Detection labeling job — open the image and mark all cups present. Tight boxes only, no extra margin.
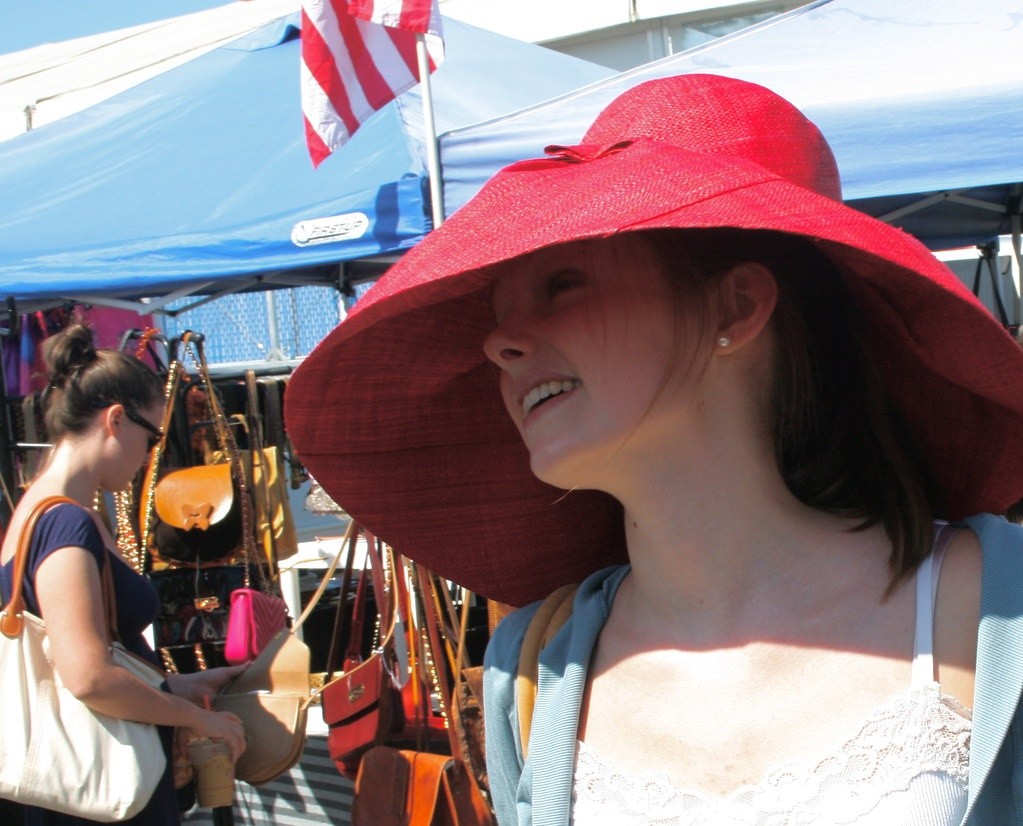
[185,739,233,809]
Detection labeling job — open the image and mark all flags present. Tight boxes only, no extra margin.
[300,0,446,169]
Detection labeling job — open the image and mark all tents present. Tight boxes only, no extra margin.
[3,8,619,665]
[436,4,1022,259]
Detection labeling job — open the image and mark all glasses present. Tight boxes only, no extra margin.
[92,400,163,455]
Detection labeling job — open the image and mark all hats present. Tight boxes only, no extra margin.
[282,72,1023,611]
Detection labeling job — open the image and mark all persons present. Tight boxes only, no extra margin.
[0,323,259,826]
[482,76,1023,826]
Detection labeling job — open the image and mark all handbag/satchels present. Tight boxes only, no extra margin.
[89,329,497,826]
[0,495,169,824]
